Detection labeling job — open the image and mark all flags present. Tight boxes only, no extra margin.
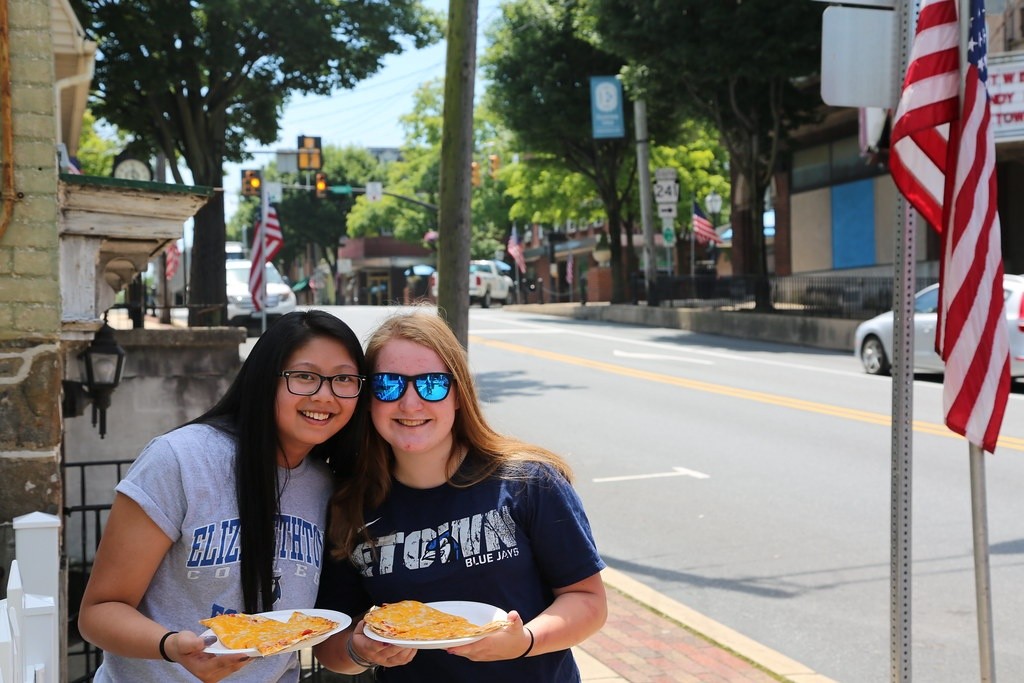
[507,216,526,275]
[166,240,181,280]
[566,247,574,285]
[691,197,724,245]
[248,167,283,311]
[889,1,1011,456]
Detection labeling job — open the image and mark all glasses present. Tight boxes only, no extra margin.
[372,371,455,403]
[275,364,367,399]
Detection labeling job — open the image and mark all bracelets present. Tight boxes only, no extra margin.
[518,625,535,660]
[155,630,180,663]
[345,632,379,670]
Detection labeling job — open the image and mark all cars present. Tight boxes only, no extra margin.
[853,274,1024,392]
[225,258,297,326]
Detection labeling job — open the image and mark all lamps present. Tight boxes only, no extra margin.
[62,312,125,439]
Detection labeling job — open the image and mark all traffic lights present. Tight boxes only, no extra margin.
[315,173,327,198]
[242,170,261,195]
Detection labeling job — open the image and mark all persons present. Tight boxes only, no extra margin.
[313,313,608,683]
[77,310,371,683]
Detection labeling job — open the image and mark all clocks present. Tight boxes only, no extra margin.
[112,158,154,181]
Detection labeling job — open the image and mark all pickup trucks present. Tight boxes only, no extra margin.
[430,260,515,308]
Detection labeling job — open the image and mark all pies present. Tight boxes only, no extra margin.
[364,600,514,640]
[199,611,339,659]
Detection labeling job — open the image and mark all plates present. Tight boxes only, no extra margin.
[199,609,352,657]
[362,601,507,649]
